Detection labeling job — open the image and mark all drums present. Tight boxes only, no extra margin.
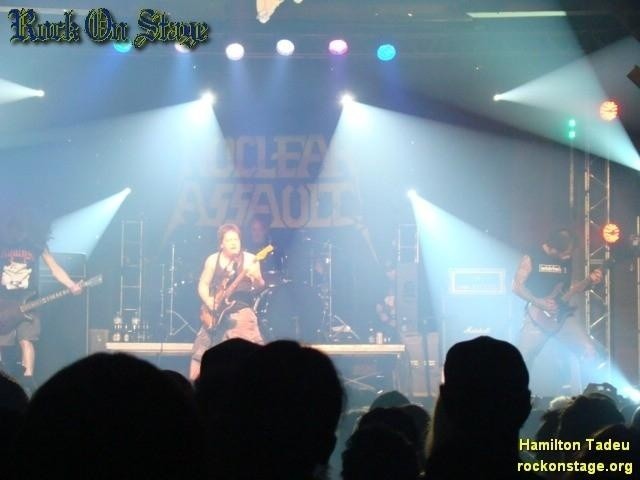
[254,280,325,341]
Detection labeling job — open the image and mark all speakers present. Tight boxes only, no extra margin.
[34,280,88,385]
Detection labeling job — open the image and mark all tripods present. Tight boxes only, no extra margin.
[158,258,361,343]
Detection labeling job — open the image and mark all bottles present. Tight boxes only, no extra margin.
[130,311,140,331]
[112,311,124,330]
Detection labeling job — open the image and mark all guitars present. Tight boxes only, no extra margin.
[201,245,273,330]
[0,273,103,336]
[527,256,616,335]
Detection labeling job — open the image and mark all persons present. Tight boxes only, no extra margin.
[3,333,638,479]
[512,228,606,384]
[189,223,266,381]
[372,261,398,393]
[0,205,83,377]
